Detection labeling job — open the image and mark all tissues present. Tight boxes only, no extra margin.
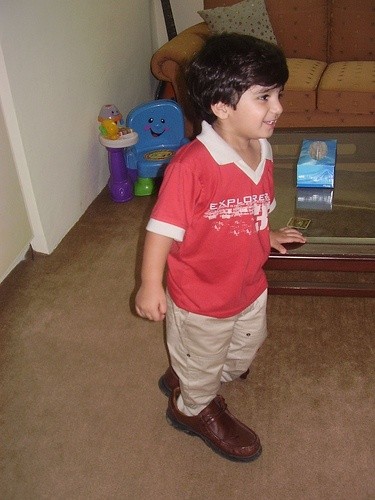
[296,138,337,188]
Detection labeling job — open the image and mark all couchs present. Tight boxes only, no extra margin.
[149,0,375,138]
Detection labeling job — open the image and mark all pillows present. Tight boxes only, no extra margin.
[197,0,278,46]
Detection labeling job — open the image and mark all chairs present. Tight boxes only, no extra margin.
[123,98,193,196]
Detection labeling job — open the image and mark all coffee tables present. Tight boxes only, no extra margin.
[240,128,375,380]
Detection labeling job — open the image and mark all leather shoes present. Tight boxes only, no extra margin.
[166,385,263,461]
[159,369,183,395]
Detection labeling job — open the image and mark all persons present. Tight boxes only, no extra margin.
[135,33,307,462]
